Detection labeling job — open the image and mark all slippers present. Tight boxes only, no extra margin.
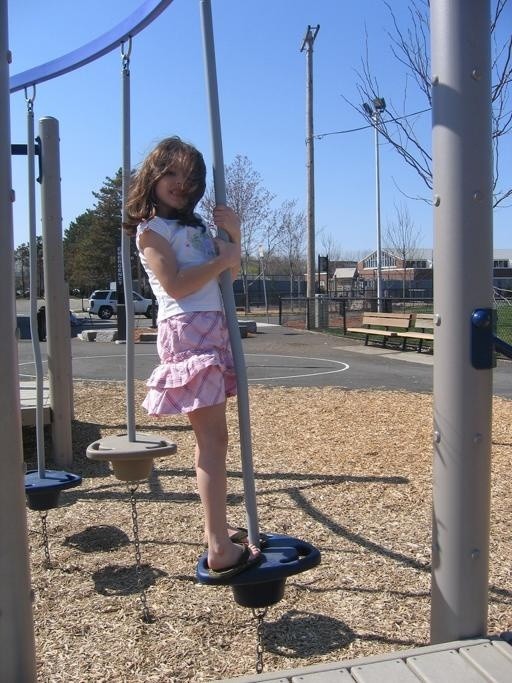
[204,526,269,579]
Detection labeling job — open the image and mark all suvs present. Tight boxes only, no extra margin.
[86,287,159,319]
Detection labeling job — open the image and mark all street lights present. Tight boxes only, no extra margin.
[363,96,387,314]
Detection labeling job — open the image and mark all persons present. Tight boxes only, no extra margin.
[120,135,269,579]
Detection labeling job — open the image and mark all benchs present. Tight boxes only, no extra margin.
[346,312,412,351]
[397,314,434,353]
